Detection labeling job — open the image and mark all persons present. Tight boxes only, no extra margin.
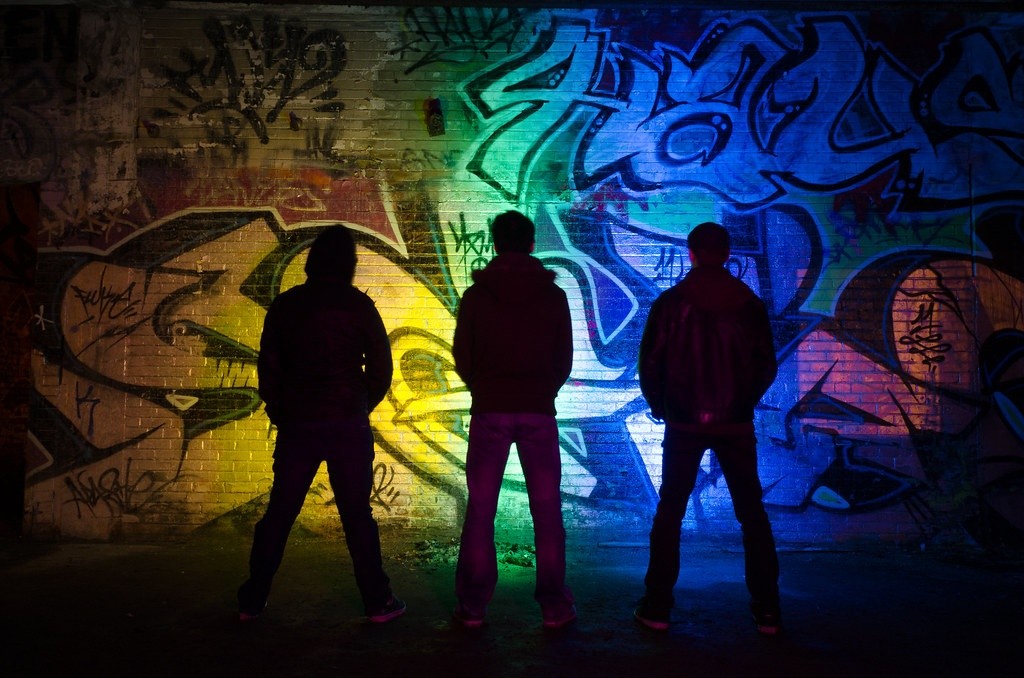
[451,209,578,631]
[633,222,786,635]
[236,222,407,623]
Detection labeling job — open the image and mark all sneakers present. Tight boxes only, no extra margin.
[368,595,407,624]
[237,601,267,622]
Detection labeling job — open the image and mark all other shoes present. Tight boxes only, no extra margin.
[453,611,484,628]
[753,615,782,633]
[633,601,669,631]
[543,605,576,628]
